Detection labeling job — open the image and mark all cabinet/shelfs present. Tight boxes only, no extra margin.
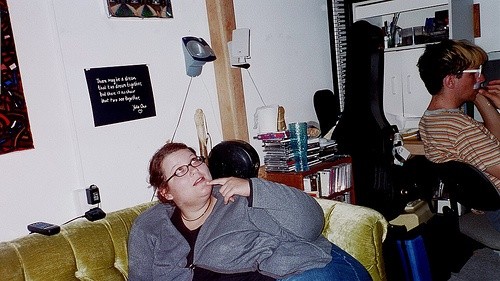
[352,0,474,132]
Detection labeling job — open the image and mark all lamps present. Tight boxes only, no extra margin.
[182,36,217,78]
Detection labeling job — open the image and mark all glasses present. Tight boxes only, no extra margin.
[458,65,483,78]
[164,156,206,184]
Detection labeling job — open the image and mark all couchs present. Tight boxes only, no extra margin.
[0,196,388,281]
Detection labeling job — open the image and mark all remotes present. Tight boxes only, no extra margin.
[27,222,61,236]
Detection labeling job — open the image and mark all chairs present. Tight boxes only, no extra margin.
[416,154,500,250]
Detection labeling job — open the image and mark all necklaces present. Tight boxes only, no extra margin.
[181,197,211,221]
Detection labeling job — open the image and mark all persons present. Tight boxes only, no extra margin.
[416,39,500,234]
[127,142,373,281]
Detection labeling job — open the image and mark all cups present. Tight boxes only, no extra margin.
[289,122,310,172]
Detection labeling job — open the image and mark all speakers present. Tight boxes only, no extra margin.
[232,28,250,68]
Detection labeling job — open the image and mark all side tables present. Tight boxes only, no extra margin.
[267,154,355,204]
